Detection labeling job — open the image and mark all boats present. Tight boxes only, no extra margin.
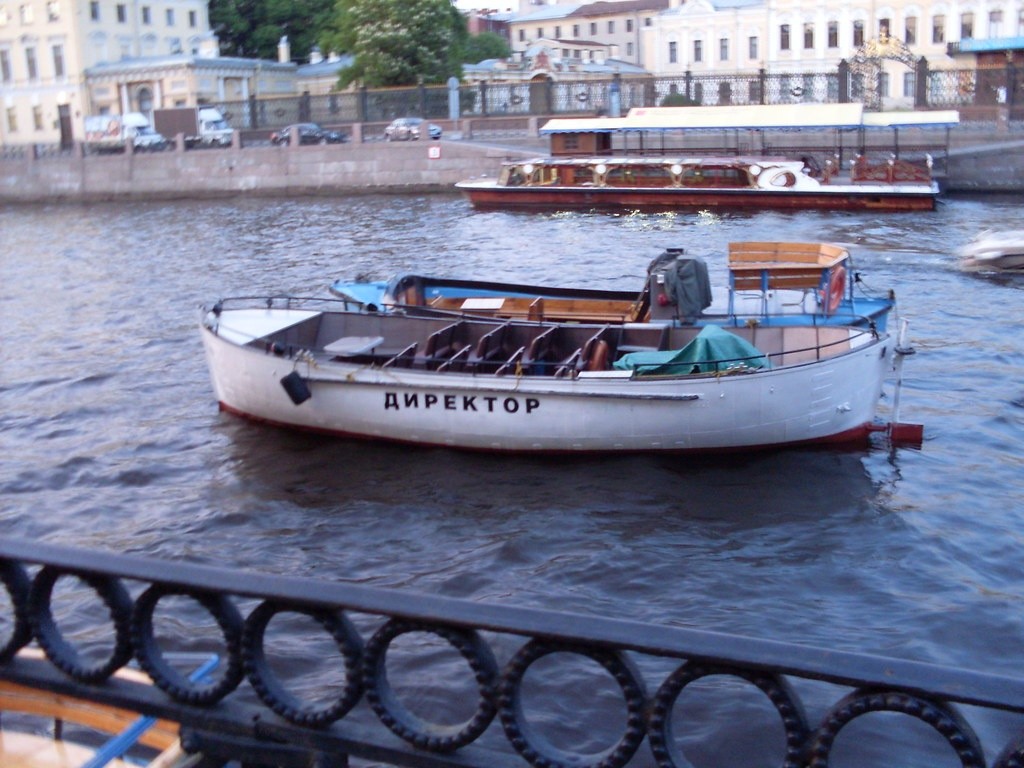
[195,296,923,450]
[328,240,896,333]
[453,104,960,212]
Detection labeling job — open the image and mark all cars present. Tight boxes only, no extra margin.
[272,124,346,147]
[385,117,442,141]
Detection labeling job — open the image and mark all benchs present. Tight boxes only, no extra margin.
[528,296,545,321]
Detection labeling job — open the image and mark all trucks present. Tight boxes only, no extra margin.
[84,108,235,150]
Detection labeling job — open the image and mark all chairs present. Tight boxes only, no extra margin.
[382,320,670,378]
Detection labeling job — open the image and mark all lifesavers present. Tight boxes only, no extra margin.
[817,266,846,311]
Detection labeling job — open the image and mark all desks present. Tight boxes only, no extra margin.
[460,298,506,317]
[324,335,384,365]
